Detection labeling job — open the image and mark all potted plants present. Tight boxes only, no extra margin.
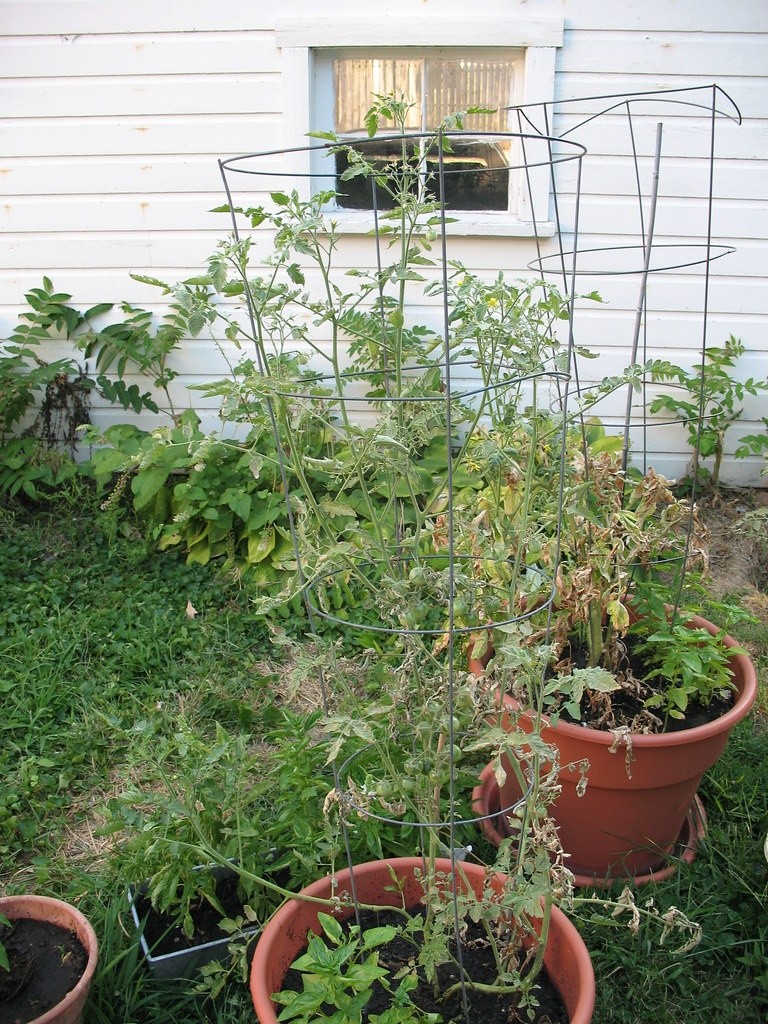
[467,340,768,890]
[0,893,100,1024]
[130,87,599,1024]
[88,705,424,985]
